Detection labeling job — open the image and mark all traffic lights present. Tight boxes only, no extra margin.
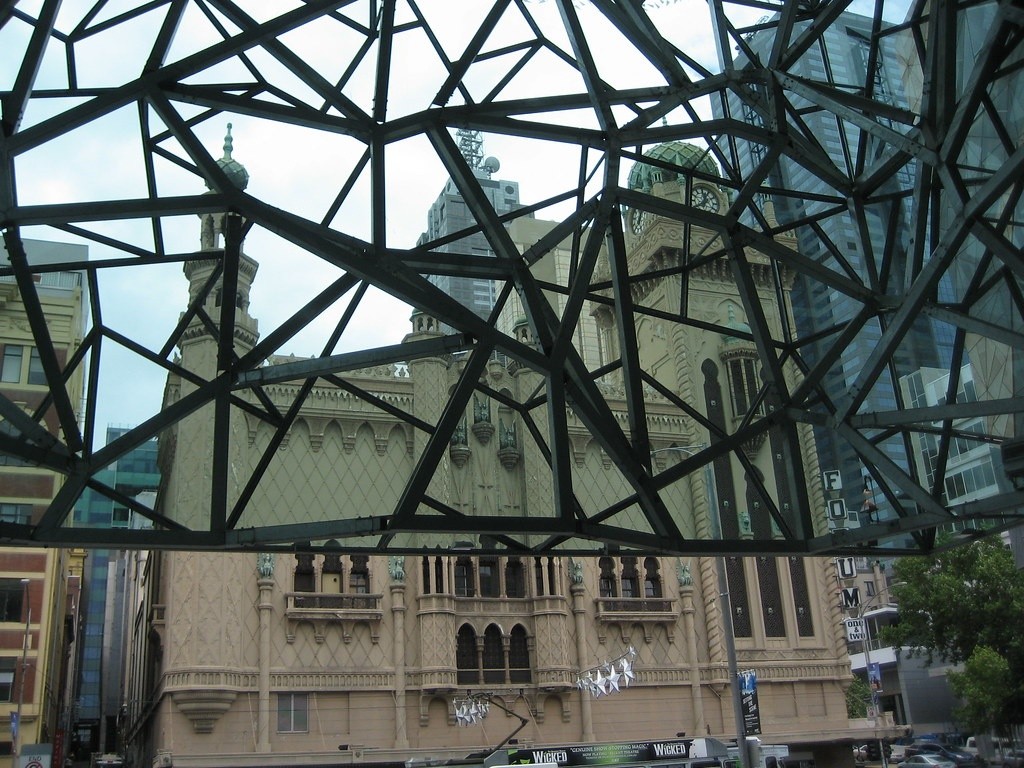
[883,740,892,759]
[866,740,879,761]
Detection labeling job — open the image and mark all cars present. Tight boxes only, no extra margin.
[853,744,906,763]
[897,753,957,768]
[966,739,1024,768]
[904,743,984,768]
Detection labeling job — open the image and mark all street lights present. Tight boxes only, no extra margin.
[857,580,908,768]
[10,578,34,768]
